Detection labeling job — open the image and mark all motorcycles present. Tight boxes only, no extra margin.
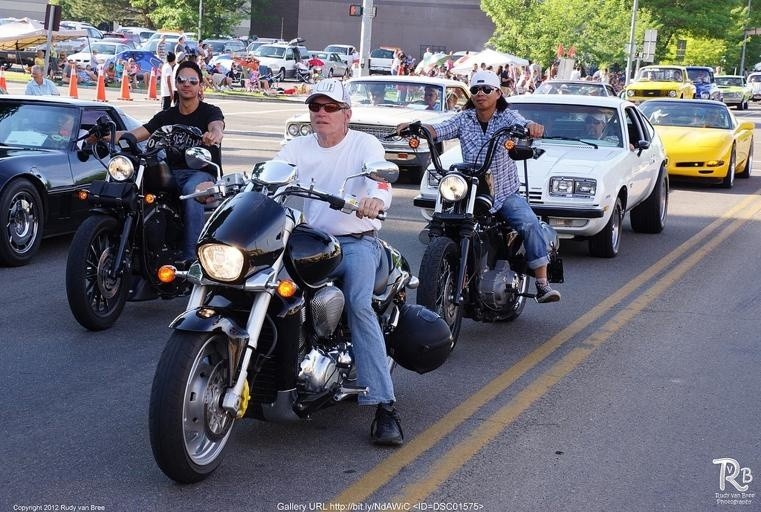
[63,116,254,331]
[382,116,564,354]
[146,136,455,485]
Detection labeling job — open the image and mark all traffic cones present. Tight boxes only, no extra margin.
[145,67,159,99]
[116,70,134,101]
[0,66,6,89]
[93,66,109,103]
[68,64,79,96]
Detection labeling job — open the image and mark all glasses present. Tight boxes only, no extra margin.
[176,75,198,85]
[308,103,346,112]
[587,119,600,124]
[470,85,493,95]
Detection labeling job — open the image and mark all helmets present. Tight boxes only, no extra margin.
[385,303,452,375]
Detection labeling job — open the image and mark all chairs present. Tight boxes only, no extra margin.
[607,124,617,135]
[80,123,96,132]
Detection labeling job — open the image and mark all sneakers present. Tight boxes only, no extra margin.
[373,406,402,446]
[535,281,560,303]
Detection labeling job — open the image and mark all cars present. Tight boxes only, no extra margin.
[413,95,673,258]
[523,64,761,188]
[281,68,474,186]
[0,17,411,89]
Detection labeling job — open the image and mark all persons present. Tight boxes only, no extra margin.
[24,34,609,447]
[707,108,720,122]
[674,71,680,80]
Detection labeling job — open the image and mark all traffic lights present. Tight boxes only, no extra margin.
[350,5,361,15]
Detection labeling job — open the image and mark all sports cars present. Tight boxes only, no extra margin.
[0,94,148,268]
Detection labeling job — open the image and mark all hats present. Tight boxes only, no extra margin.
[304,79,350,107]
[587,110,606,124]
[175,61,202,82]
[470,70,499,89]
